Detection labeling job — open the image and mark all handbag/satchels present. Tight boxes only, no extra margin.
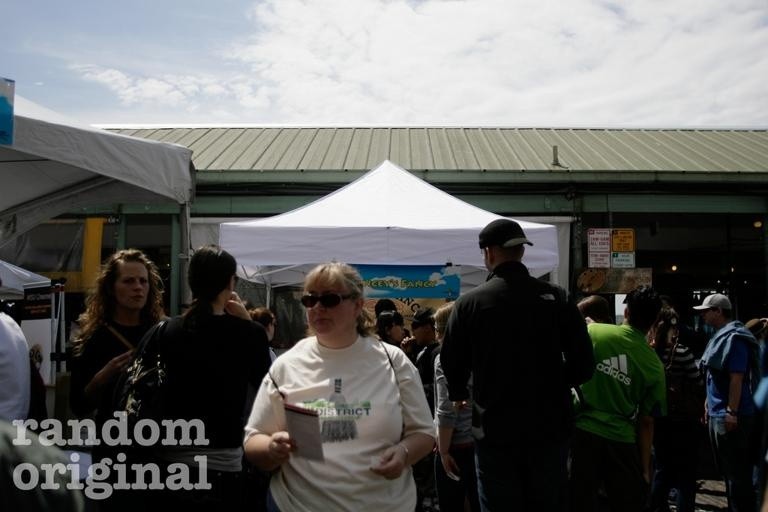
[114,353,169,422]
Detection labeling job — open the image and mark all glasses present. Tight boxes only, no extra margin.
[301,293,351,308]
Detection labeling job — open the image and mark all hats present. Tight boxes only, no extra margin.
[403,307,436,323]
[479,220,533,248]
[745,318,768,335]
[693,293,732,310]
